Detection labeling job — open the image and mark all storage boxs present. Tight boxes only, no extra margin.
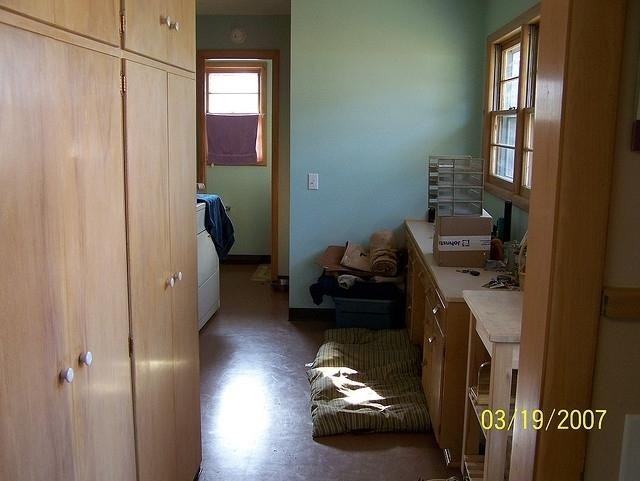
[332,296,395,330]
[433,208,493,267]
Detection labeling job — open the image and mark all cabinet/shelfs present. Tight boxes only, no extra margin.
[461,290,524,481]
[0,0,201,481]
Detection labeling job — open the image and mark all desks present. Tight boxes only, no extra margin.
[403,220,520,468]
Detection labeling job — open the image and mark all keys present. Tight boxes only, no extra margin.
[456,267,480,277]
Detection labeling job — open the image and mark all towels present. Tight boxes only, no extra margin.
[206,114,258,165]
